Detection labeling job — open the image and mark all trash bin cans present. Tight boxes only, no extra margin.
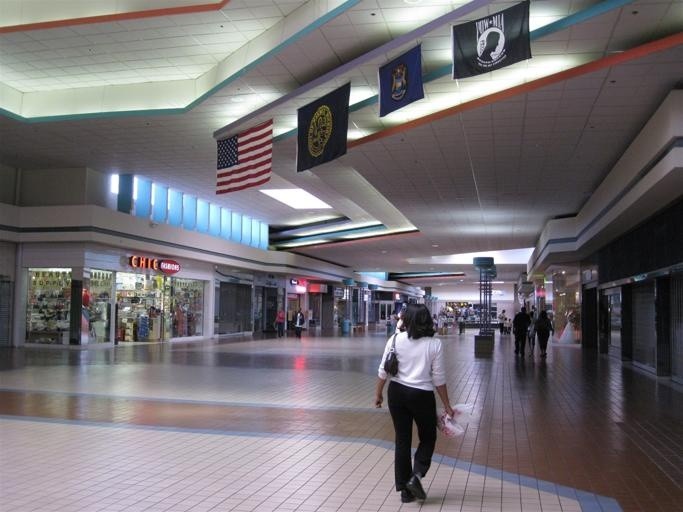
[387,324,395,338]
[459,322,466,336]
[341,320,350,337]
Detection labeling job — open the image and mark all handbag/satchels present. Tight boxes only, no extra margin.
[384,333,398,376]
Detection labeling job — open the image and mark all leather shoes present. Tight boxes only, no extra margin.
[401,475,427,503]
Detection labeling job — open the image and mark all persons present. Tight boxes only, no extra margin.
[149,306,160,317]
[498,310,512,335]
[294,308,304,339]
[373,303,454,503]
[513,307,554,357]
[275,308,285,337]
